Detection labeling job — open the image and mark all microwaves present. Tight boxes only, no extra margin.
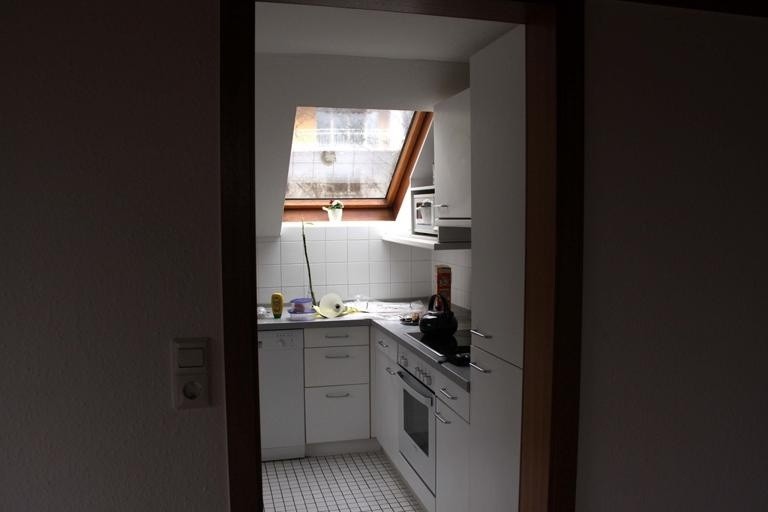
[411,192,439,238]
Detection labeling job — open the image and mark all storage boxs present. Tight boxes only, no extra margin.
[434,264,452,310]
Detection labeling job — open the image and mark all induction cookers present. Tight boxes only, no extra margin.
[405,327,471,357]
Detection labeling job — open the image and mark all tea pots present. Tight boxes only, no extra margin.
[420,294,460,339]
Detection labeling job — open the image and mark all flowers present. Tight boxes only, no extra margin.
[322,199,345,210]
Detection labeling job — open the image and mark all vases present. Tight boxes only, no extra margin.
[327,209,343,223]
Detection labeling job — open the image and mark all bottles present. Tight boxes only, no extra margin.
[397,301,424,326]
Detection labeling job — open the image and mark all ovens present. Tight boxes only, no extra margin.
[393,363,437,511]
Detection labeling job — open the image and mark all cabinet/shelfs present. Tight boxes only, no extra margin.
[431,89,471,232]
[253,326,305,463]
[434,373,470,511]
[370,328,399,465]
[303,322,370,448]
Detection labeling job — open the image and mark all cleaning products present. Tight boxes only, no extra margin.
[271,293,284,318]
